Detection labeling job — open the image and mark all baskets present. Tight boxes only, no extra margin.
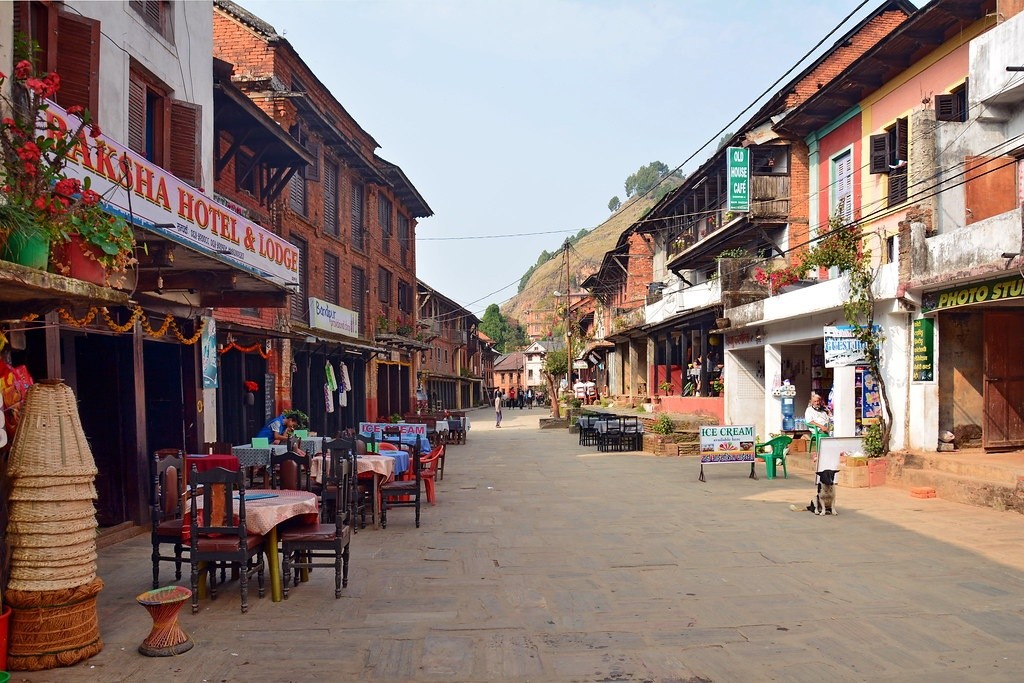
[7,572,96,591]
[9,499,97,522]
[6,515,99,534]
[13,551,97,567]
[7,528,96,547]
[9,482,98,500]
[12,539,96,561]
[10,561,97,581]
[11,475,96,487]
[6,383,98,476]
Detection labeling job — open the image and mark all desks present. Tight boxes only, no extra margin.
[378,449,408,480]
[593,420,645,451]
[375,436,431,454]
[310,455,397,530]
[576,416,599,444]
[299,436,332,454]
[186,455,239,485]
[182,489,319,601]
[232,443,287,489]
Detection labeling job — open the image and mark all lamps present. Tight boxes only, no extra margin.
[407,350,426,363]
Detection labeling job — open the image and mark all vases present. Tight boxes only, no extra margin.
[1,231,50,272]
[378,328,387,334]
[666,390,672,396]
[653,398,660,404]
[52,233,108,286]
[719,392,724,397]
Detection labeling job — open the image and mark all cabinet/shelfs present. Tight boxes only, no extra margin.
[810,344,834,391]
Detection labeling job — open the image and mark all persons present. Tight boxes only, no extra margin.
[494,386,507,428]
[560,378,568,395]
[572,378,596,399]
[804,395,834,436]
[527,386,534,410]
[509,387,515,411]
[517,386,524,410]
[256,415,298,446]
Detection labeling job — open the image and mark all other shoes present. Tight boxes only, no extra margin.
[496,425,501,428]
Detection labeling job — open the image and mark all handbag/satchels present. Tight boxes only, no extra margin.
[532,396,535,400]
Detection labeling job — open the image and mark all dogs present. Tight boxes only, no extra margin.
[790,470,840,516]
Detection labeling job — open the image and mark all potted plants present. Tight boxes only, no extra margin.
[864,422,886,488]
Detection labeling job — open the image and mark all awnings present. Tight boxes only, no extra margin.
[580,242,631,306]
[214,74,318,208]
[665,215,788,286]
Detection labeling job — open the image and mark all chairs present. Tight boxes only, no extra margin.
[151,408,471,614]
[751,436,793,479]
[804,420,828,454]
[577,386,597,404]
[579,410,639,451]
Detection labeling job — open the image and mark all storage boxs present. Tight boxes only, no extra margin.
[838,465,869,488]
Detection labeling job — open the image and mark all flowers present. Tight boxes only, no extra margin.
[0,29,150,287]
[376,307,424,341]
[652,379,674,398]
[711,376,725,391]
